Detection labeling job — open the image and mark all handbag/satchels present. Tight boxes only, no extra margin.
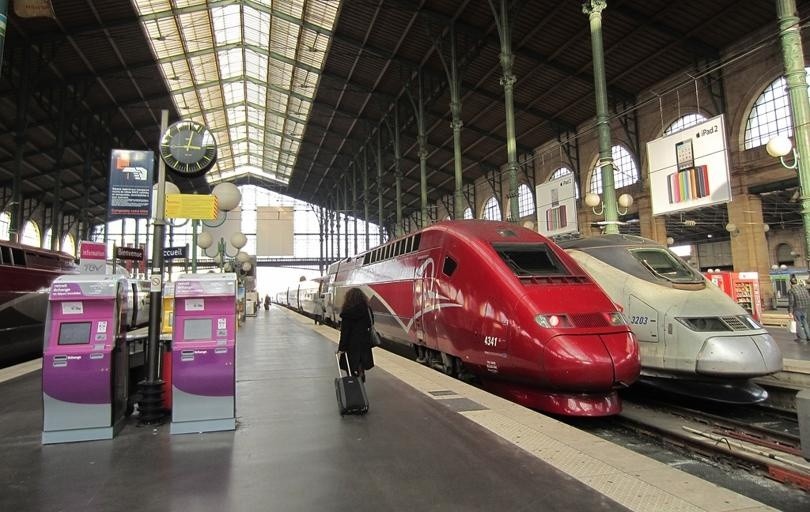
[322,306,326,313]
[368,307,381,348]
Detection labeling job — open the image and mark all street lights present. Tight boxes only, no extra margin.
[151,182,243,274]
[214,250,252,280]
[195,230,248,273]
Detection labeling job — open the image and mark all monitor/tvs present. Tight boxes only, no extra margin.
[58,321,92,344]
[184,319,212,340]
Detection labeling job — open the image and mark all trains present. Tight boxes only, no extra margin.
[265,219,641,418]
[550,234,783,405]
[0,239,167,369]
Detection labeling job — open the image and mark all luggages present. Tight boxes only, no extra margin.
[264,304,269,310]
[332,351,369,417]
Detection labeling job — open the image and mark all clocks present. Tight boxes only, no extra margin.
[157,120,219,177]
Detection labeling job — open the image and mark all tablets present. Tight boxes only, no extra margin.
[674,138,694,172]
[550,188,559,207]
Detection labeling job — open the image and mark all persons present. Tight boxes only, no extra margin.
[313,295,327,326]
[787,277,810,342]
[337,288,374,383]
[265,293,272,309]
